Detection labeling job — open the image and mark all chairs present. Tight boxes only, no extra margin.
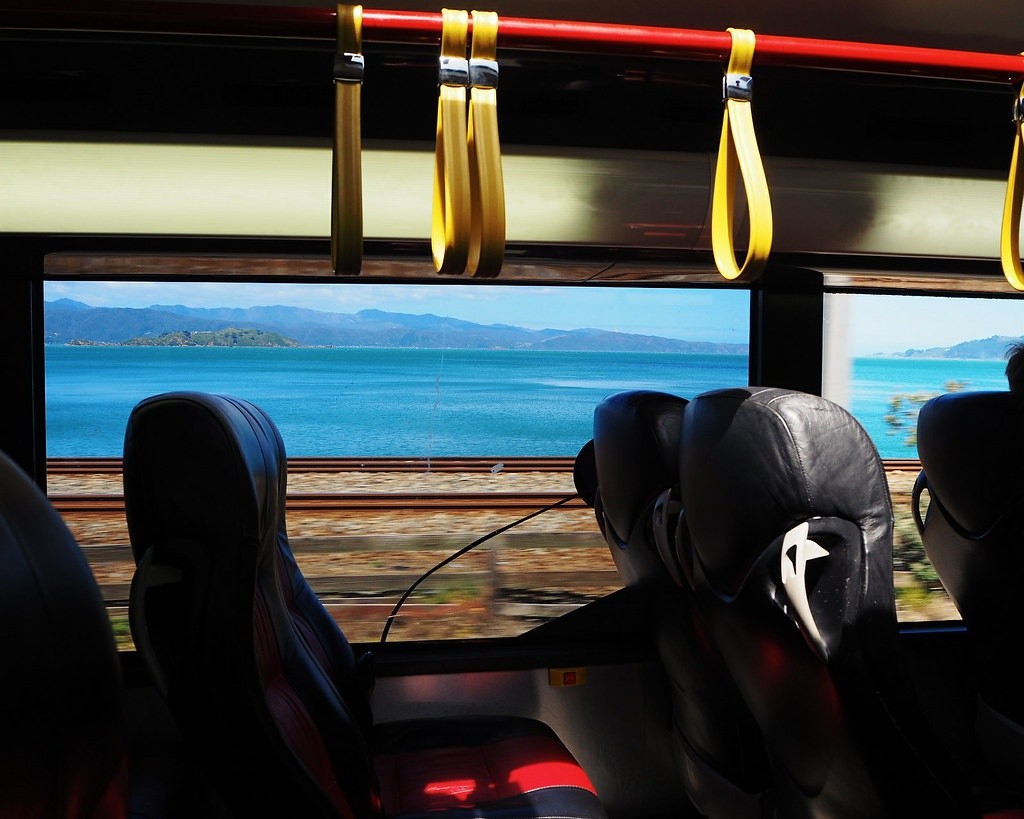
[0,390,1024,819]
